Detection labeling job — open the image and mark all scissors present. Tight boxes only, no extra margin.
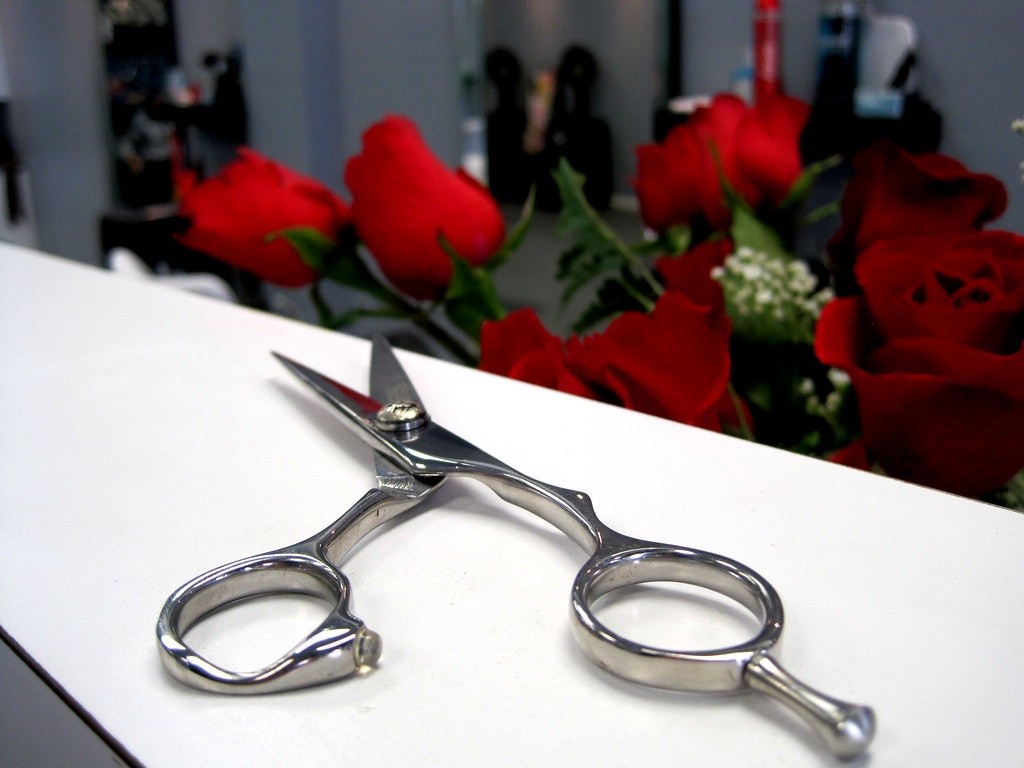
[156,329,877,763]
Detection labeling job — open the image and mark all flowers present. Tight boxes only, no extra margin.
[182,84,1024,523]
